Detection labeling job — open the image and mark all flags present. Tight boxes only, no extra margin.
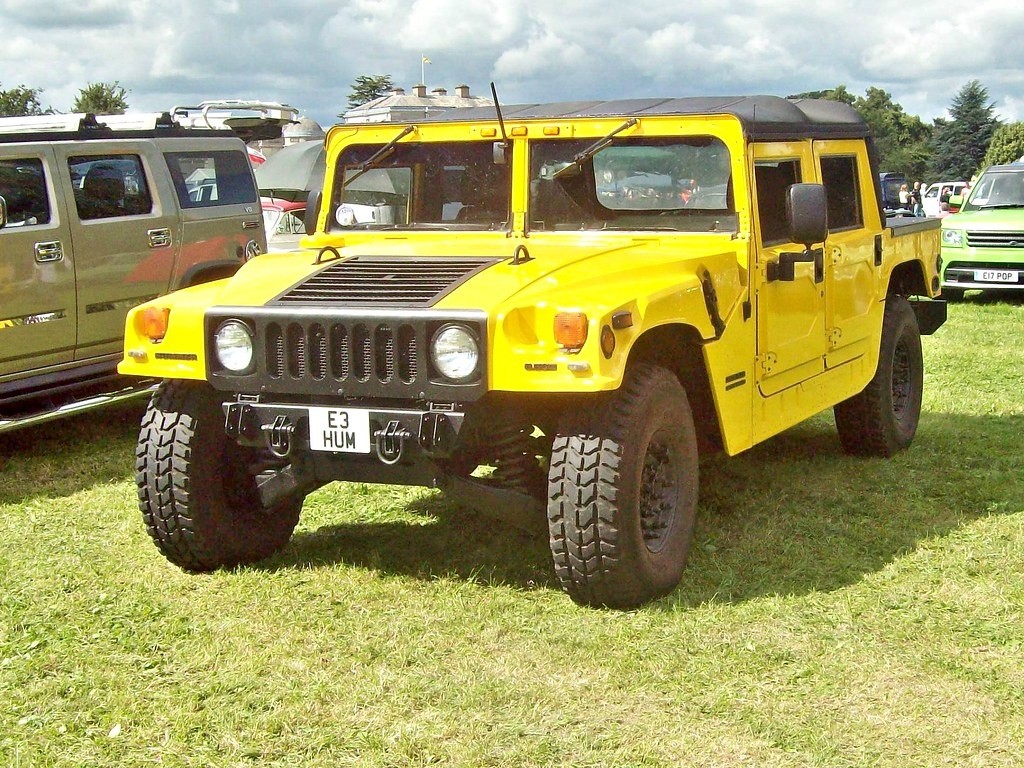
[423,57,432,63]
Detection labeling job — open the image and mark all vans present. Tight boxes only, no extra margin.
[880,172,975,218]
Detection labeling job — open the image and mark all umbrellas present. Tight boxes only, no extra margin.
[254,139,412,203]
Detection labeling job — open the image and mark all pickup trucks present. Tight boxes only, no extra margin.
[114,100,946,612]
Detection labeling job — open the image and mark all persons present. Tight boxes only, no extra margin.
[899,184,913,210]
[961,181,972,202]
[690,179,701,193]
[911,182,926,217]
[940,189,949,203]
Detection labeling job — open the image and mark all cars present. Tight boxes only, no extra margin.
[938,156,1024,303]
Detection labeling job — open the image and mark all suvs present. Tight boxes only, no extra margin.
[0,103,409,426]
